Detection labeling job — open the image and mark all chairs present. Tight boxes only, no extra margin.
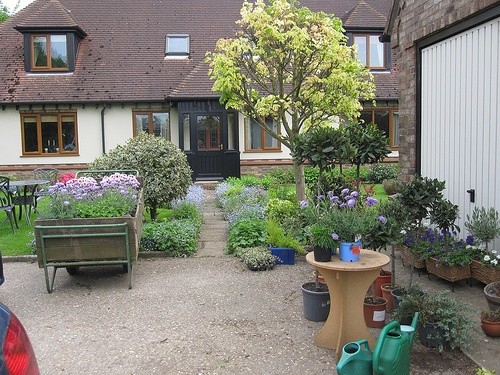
[0,175,19,234]
[33,167,58,213]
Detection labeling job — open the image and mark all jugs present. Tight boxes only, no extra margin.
[335,339,373,375]
[372,312,419,375]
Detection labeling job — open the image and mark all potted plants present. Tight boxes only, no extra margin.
[281,119,393,322]
[363,215,407,329]
[389,286,488,354]
[385,172,462,325]
[262,218,307,265]
[480,306,500,338]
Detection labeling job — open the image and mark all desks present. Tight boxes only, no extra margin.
[306,248,390,365]
[0,180,49,227]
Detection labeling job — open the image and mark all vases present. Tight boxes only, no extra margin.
[338,240,363,262]
[312,243,333,263]
[32,169,146,295]
[468,257,500,288]
[424,254,472,294]
[363,297,388,328]
[370,269,391,298]
[391,241,426,277]
[483,280,500,312]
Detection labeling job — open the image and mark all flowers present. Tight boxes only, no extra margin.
[296,183,389,249]
[394,198,500,269]
[34,172,142,220]
